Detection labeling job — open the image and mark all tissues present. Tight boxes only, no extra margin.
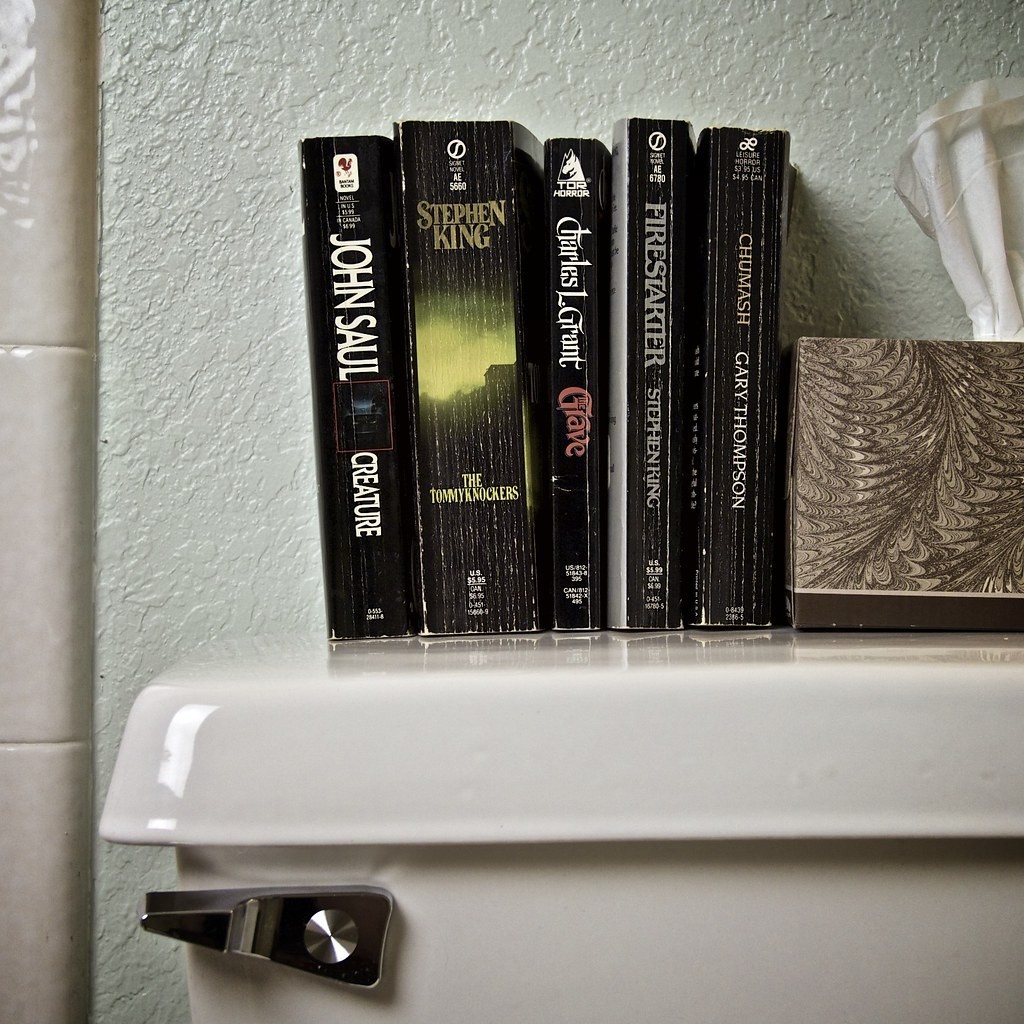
[781,76,1024,633]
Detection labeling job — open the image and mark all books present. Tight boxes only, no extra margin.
[302,117,791,640]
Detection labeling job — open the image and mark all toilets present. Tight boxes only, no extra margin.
[91,630,1024,1024]
